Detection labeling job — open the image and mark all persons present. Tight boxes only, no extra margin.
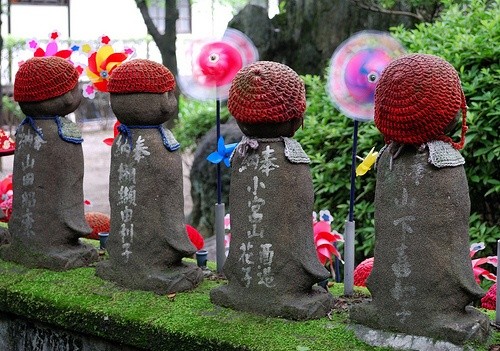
[209,59,336,321]
[94,58,205,295]
[8,56,99,271]
[348,53,490,346]
